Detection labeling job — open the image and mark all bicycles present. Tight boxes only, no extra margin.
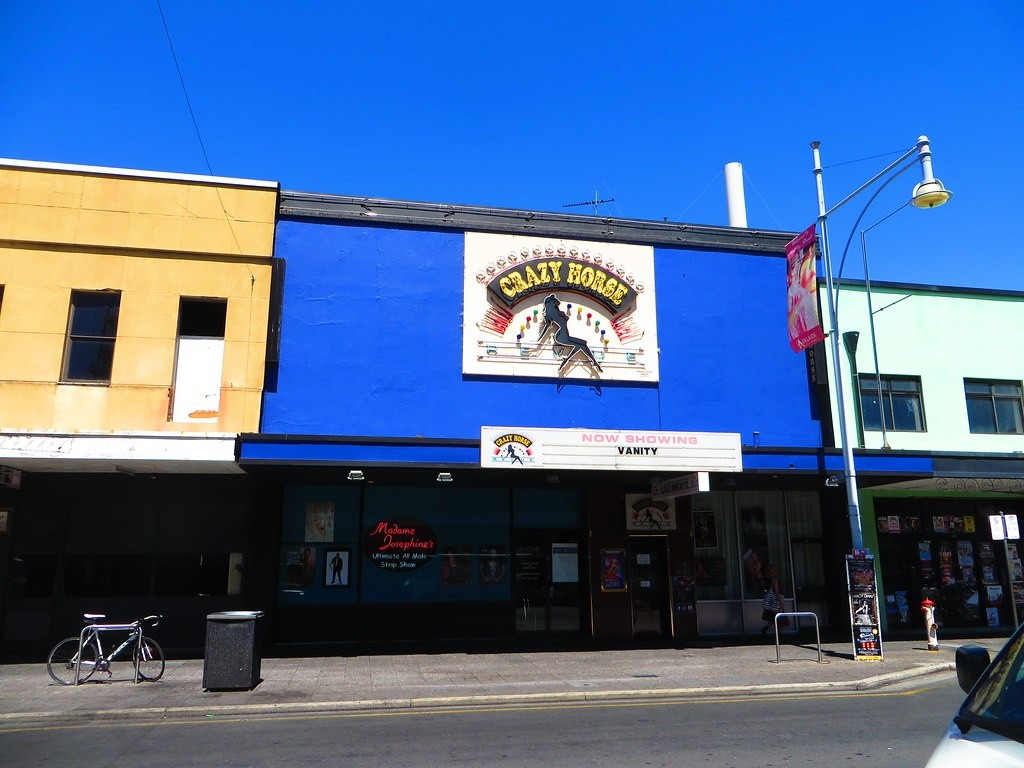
[44,612,168,684]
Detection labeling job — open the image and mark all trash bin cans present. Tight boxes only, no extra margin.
[202,611,264,689]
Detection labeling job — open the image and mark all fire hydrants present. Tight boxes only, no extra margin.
[917,597,941,651]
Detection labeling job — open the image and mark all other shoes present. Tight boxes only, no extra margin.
[761,628,768,638]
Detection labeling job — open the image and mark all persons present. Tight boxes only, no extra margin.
[760,566,790,641]
[695,511,767,596]
[299,515,505,585]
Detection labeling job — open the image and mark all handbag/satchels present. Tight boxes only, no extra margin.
[762,584,785,613]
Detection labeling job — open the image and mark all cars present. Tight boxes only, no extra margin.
[926,621,1024,768]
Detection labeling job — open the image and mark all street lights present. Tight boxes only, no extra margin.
[807,133,950,547]
